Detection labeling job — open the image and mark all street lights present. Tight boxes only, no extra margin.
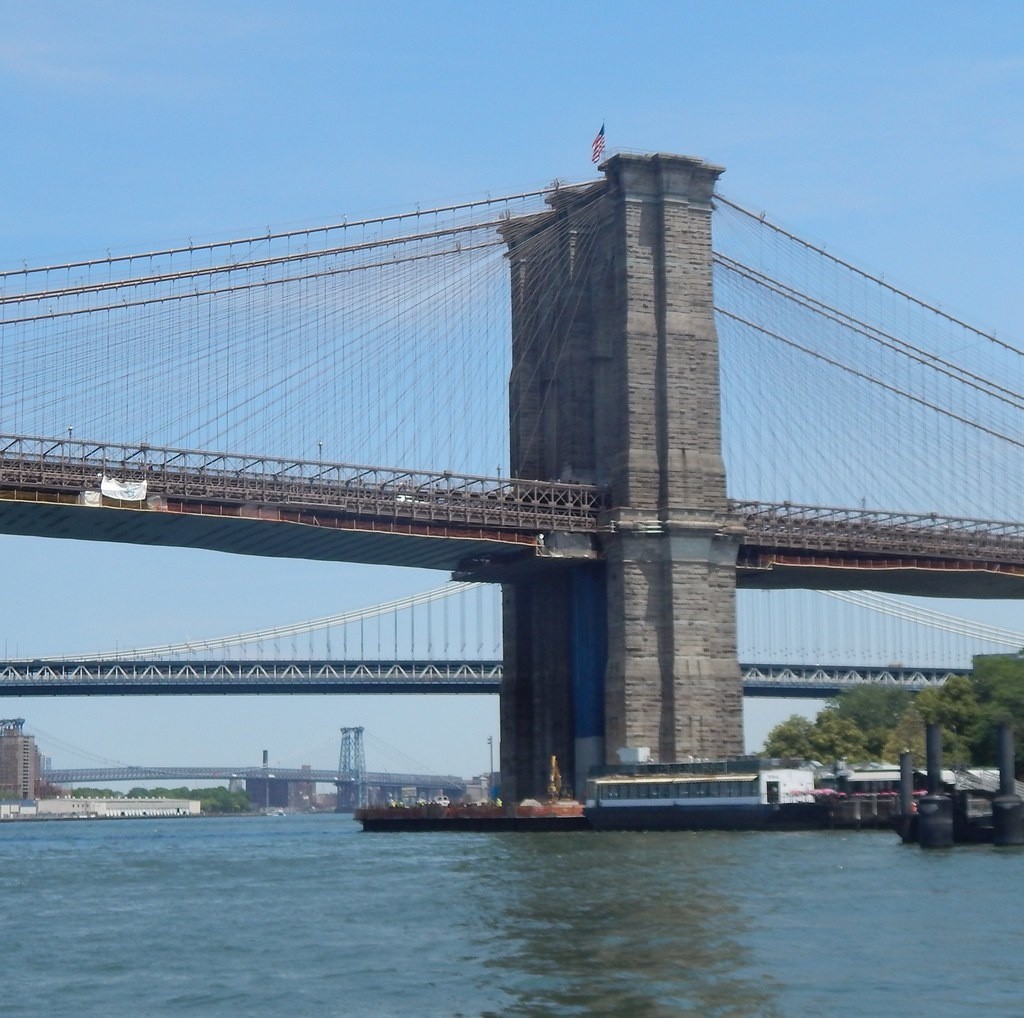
[318,442,323,484]
[68,425,75,463]
[497,465,503,488]
[861,496,867,523]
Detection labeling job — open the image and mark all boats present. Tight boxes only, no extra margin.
[580,766,840,832]
[264,808,287,817]
[351,800,589,834]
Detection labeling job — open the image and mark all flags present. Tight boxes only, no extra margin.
[592,123,604,164]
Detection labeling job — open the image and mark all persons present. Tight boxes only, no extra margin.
[137,457,144,471]
[147,460,154,471]
[770,787,778,804]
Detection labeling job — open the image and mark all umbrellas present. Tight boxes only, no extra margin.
[785,787,838,804]
[839,791,846,798]
[851,790,928,797]
